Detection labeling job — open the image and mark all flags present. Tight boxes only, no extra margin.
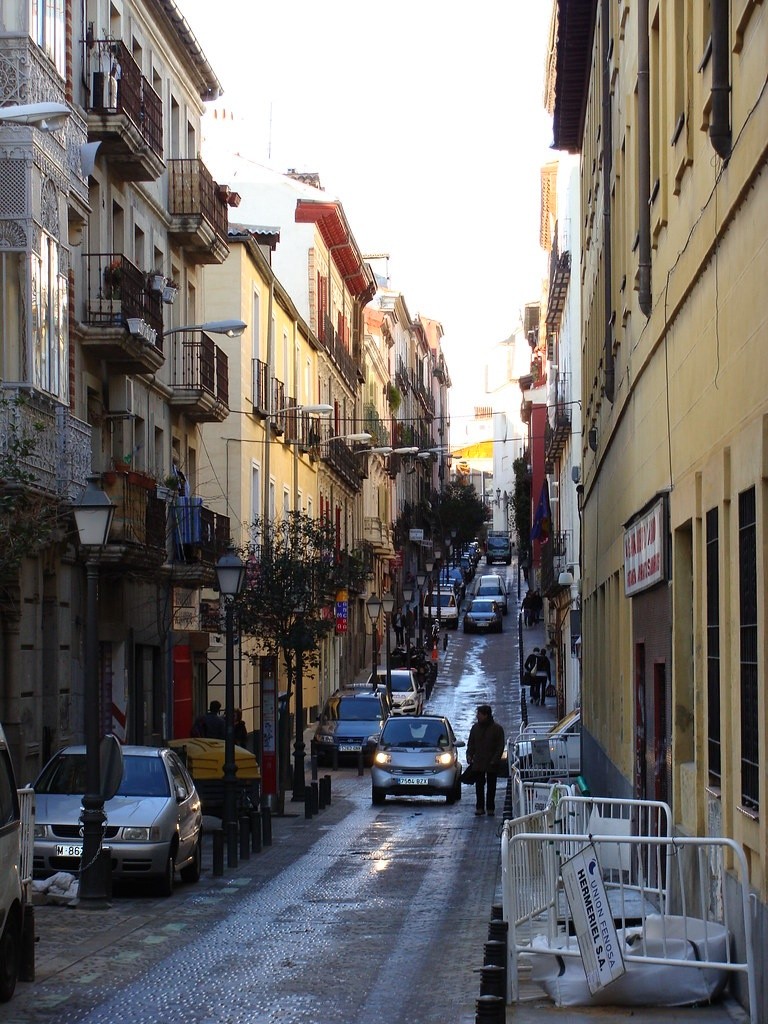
[531,478,553,542]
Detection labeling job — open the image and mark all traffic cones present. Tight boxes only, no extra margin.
[432,641,439,660]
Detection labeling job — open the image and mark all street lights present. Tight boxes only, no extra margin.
[425,558,435,645]
[366,592,382,689]
[285,559,313,802]
[402,582,414,670]
[433,547,442,627]
[450,529,456,568]
[69,473,119,909]
[381,590,395,709]
[444,537,451,583]
[416,570,427,646]
[216,547,250,868]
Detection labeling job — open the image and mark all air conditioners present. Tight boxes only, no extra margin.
[89,71,117,113]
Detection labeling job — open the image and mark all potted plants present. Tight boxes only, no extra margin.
[156,474,180,503]
[147,269,182,304]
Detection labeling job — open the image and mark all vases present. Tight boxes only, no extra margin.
[127,471,156,489]
[227,192,241,207]
[220,184,231,198]
[85,299,129,326]
[103,471,116,486]
[115,463,131,472]
[127,318,158,346]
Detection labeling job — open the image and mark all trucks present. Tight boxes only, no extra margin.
[484,530,515,565]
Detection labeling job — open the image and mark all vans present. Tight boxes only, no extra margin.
[470,575,510,615]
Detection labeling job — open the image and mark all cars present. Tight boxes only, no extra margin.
[504,712,580,783]
[18,745,202,894]
[368,715,465,804]
[0,722,22,1001]
[366,670,424,716]
[433,541,482,604]
[462,599,502,633]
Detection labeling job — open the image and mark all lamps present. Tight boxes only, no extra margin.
[418,447,448,452]
[437,453,462,459]
[162,321,248,338]
[0,101,71,133]
[417,453,430,458]
[316,433,372,444]
[265,403,333,421]
[353,447,393,457]
[382,447,419,456]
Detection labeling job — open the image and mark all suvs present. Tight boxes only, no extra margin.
[313,688,391,765]
[422,589,459,629]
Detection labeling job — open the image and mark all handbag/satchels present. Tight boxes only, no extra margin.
[523,672,531,686]
[545,682,557,697]
[531,658,537,676]
[460,763,476,785]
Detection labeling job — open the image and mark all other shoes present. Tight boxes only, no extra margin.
[541,703,545,706]
[475,809,486,815]
[536,700,539,706]
[530,697,533,704]
[488,810,495,816]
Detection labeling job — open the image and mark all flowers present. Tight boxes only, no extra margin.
[95,259,127,301]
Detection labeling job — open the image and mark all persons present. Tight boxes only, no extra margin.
[423,722,441,745]
[525,647,550,706]
[521,589,542,628]
[466,705,504,816]
[193,700,248,749]
[391,606,405,647]
